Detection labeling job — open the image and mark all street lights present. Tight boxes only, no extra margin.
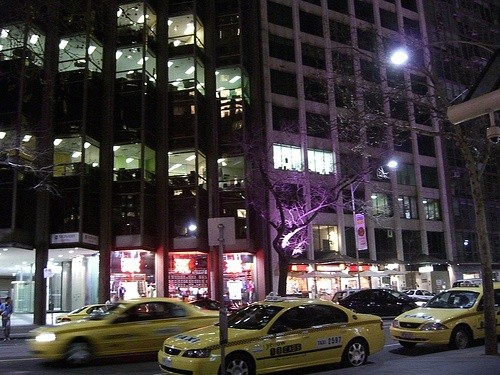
[351,160,397,289]
[390,40,495,66]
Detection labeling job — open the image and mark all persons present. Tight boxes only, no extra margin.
[0,297,14,342]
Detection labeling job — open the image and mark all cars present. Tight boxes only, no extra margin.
[337,289,418,316]
[157,292,385,375]
[332,278,482,303]
[56,304,106,324]
[389,282,500,350]
[24,297,230,366]
